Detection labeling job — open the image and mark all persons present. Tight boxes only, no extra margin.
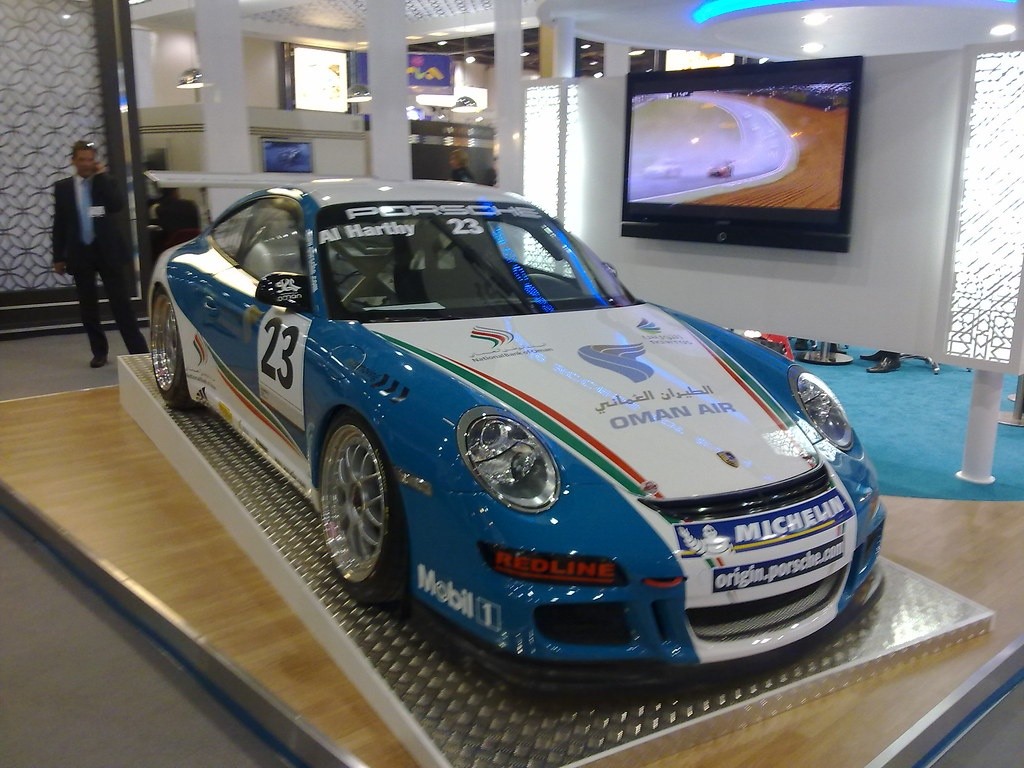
[860,350,901,373]
[447,148,479,184]
[52,140,150,369]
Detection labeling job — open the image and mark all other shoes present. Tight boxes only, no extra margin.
[90,355,107,367]
[795,343,811,350]
[831,349,846,354]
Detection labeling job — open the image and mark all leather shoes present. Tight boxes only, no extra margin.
[860,350,893,361]
[867,353,900,373]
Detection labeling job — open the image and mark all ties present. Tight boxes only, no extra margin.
[81,180,96,245]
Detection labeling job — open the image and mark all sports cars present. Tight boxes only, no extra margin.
[148,176,890,684]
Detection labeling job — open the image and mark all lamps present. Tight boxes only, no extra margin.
[347,83,374,103]
[175,37,204,90]
[451,96,479,114]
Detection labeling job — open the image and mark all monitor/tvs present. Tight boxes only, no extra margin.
[621,56,865,253]
